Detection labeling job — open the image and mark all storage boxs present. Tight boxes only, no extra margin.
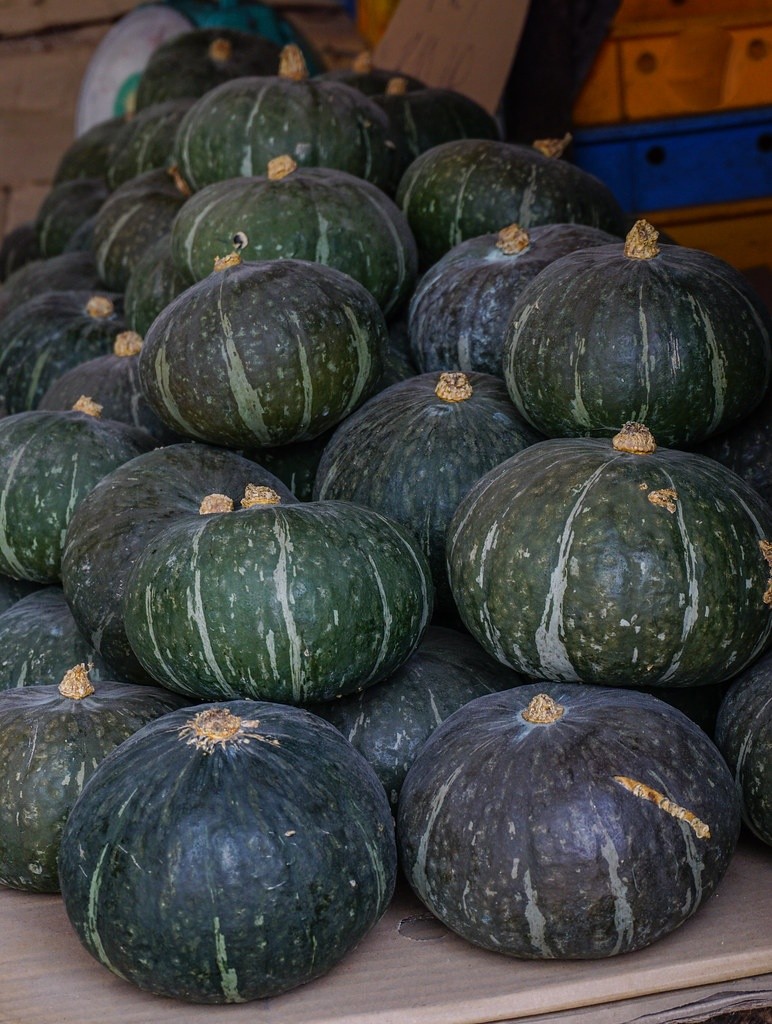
[554,0,772,271]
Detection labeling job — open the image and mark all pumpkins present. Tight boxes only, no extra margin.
[1,27,772,1005]
